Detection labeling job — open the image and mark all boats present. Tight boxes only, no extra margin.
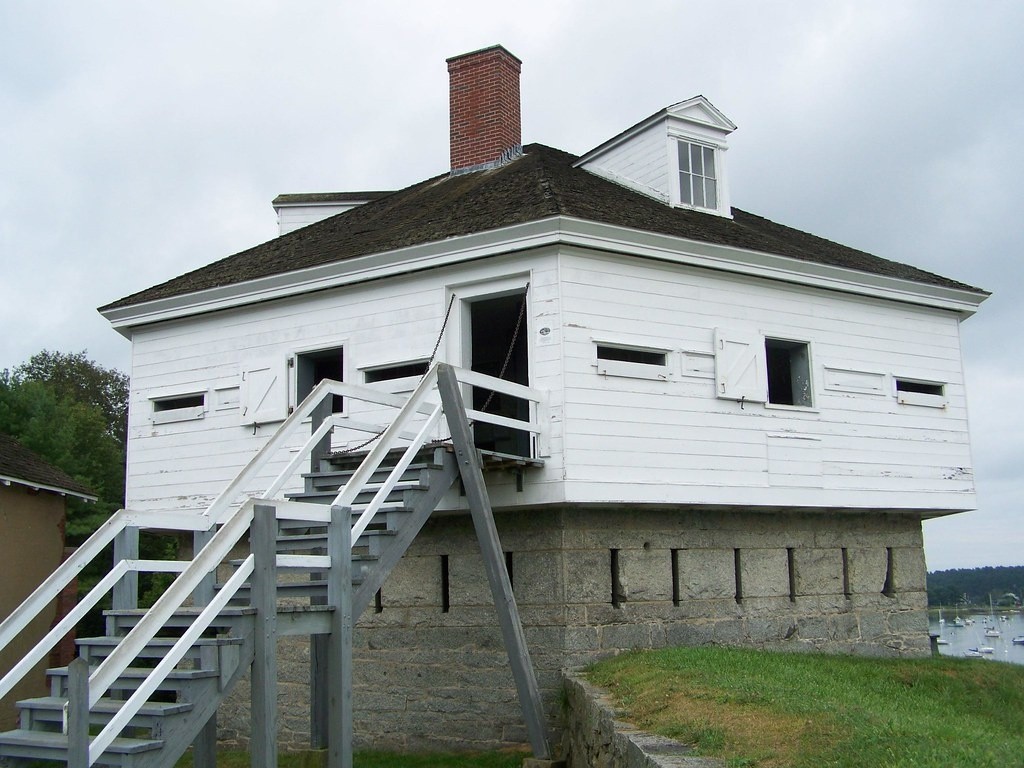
[939,602,976,627]
[982,593,1008,637]
[963,633,994,657]
[937,639,949,645]
[1012,635,1024,644]
